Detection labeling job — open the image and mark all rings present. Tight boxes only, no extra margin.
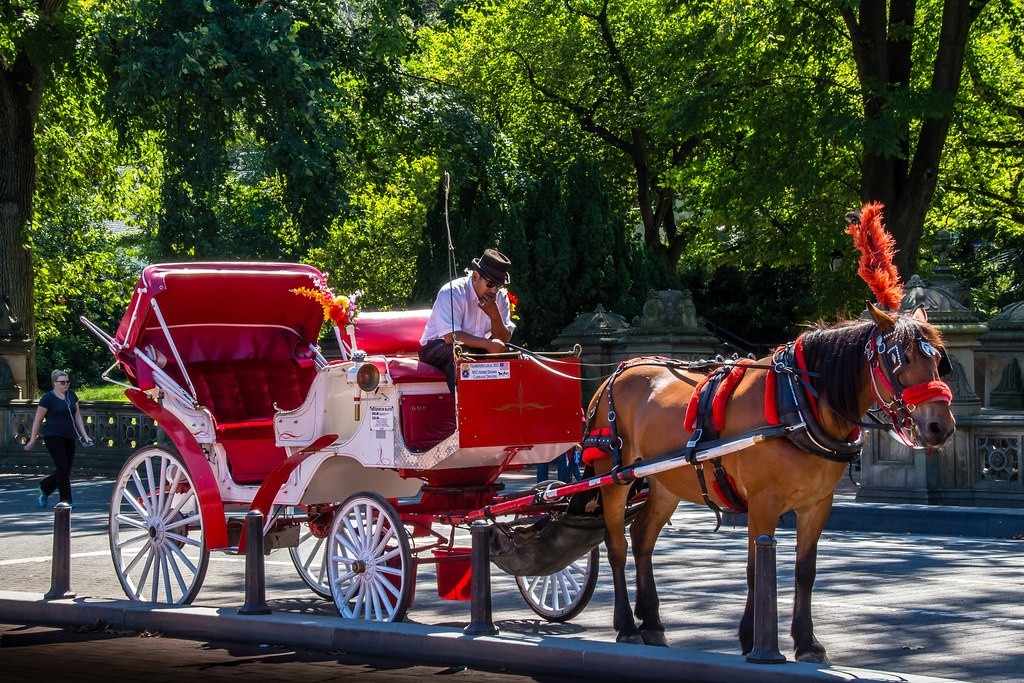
[480,302,485,306]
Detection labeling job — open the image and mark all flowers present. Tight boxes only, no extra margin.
[288,272,365,343]
[506,291,521,322]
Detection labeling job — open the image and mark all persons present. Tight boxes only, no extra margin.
[23,369,93,510]
[418,250,513,397]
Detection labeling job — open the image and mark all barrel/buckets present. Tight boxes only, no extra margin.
[432,547,472,601]
[381,558,419,607]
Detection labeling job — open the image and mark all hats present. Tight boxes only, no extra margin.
[471,248,511,284]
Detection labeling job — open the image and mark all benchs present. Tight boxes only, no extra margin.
[273,356,416,447]
[334,309,446,380]
[142,323,318,439]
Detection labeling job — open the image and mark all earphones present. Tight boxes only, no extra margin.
[55,383,58,387]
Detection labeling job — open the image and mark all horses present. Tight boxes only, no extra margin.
[580,302,957,666]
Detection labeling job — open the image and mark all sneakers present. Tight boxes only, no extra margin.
[38,484,48,508]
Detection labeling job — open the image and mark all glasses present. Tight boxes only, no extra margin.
[475,269,503,290]
[55,380,72,386]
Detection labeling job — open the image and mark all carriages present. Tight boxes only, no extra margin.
[79,260,957,664]
